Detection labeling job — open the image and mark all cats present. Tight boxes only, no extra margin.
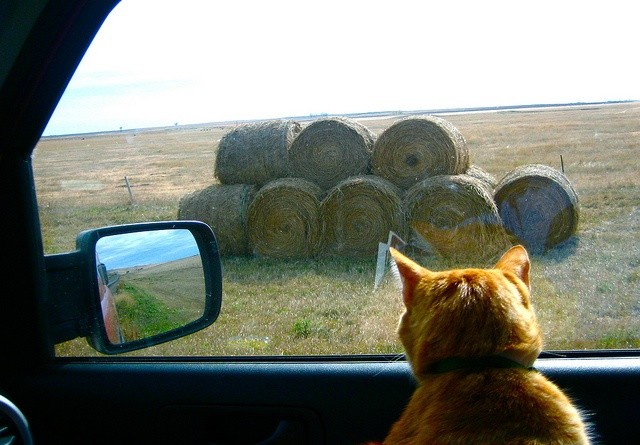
[382,244,601,445]
